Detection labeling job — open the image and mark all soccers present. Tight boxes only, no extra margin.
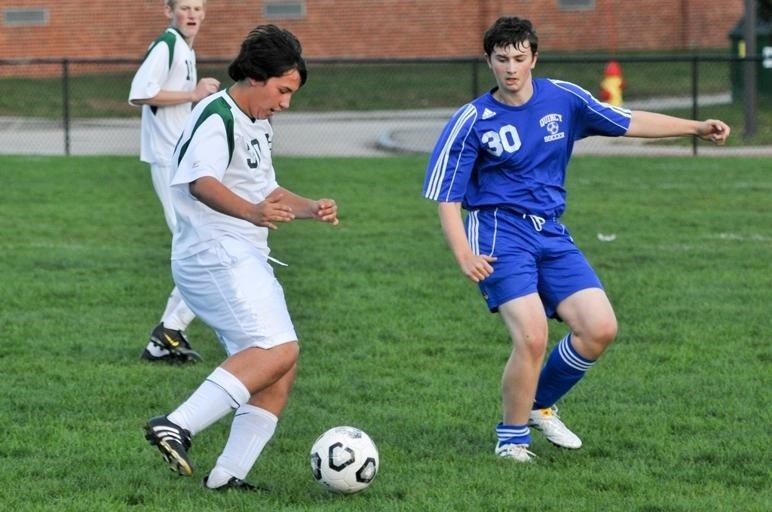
[308,426,380,496]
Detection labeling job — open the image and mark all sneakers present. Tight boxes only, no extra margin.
[529,406,582,450]
[144,415,192,476]
[203,475,264,492]
[138,323,200,365]
[494,442,528,464]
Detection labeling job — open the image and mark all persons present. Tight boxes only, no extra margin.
[127,0,220,365]
[422,16,730,461]
[145,22,339,495]
